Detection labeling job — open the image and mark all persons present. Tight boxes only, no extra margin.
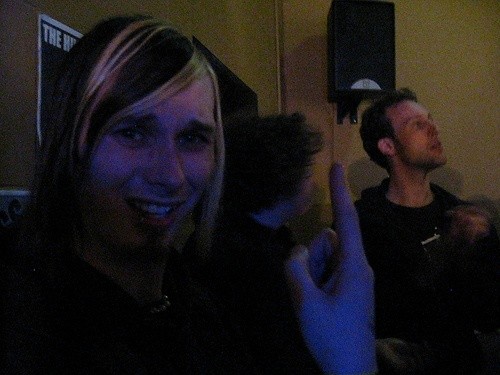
[359,88,500,375]
[0,14,378,375]
[182,114,324,374]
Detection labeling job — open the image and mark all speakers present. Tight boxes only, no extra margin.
[328,0,396,98]
